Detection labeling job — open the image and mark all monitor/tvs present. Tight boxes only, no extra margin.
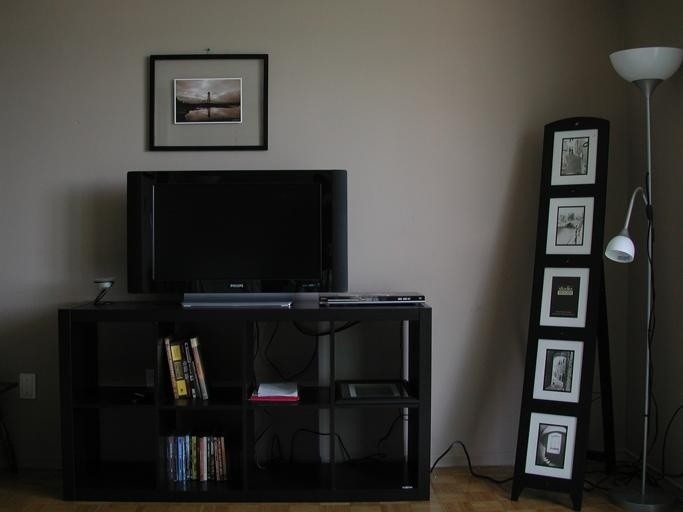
[127,170,347,310]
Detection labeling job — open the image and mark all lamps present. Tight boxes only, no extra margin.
[600,44,683,510]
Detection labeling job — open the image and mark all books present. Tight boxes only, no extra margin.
[248,382,302,402]
[164,334,229,483]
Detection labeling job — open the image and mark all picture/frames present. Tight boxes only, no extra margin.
[147,52,268,152]
[509,115,619,512]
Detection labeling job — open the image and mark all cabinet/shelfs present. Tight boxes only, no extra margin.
[53,299,434,503]
